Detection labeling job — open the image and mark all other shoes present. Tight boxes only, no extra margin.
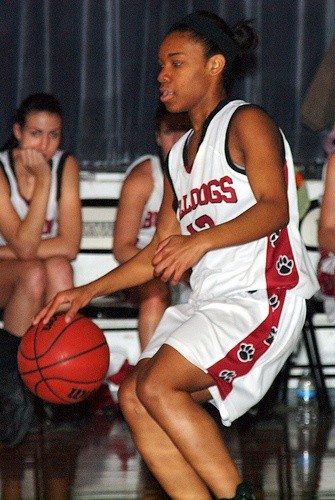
[0,372,35,448]
[223,482,260,500]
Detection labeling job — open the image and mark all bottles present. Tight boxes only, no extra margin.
[298,369,317,424]
[298,425,318,491]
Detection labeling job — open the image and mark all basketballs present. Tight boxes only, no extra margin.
[17,311,110,404]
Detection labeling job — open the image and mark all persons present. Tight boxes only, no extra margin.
[0,423,81,499]
[0,92,83,433]
[32,10,320,499]
[315,150,335,295]
[111,103,192,353]
[237,410,278,499]
